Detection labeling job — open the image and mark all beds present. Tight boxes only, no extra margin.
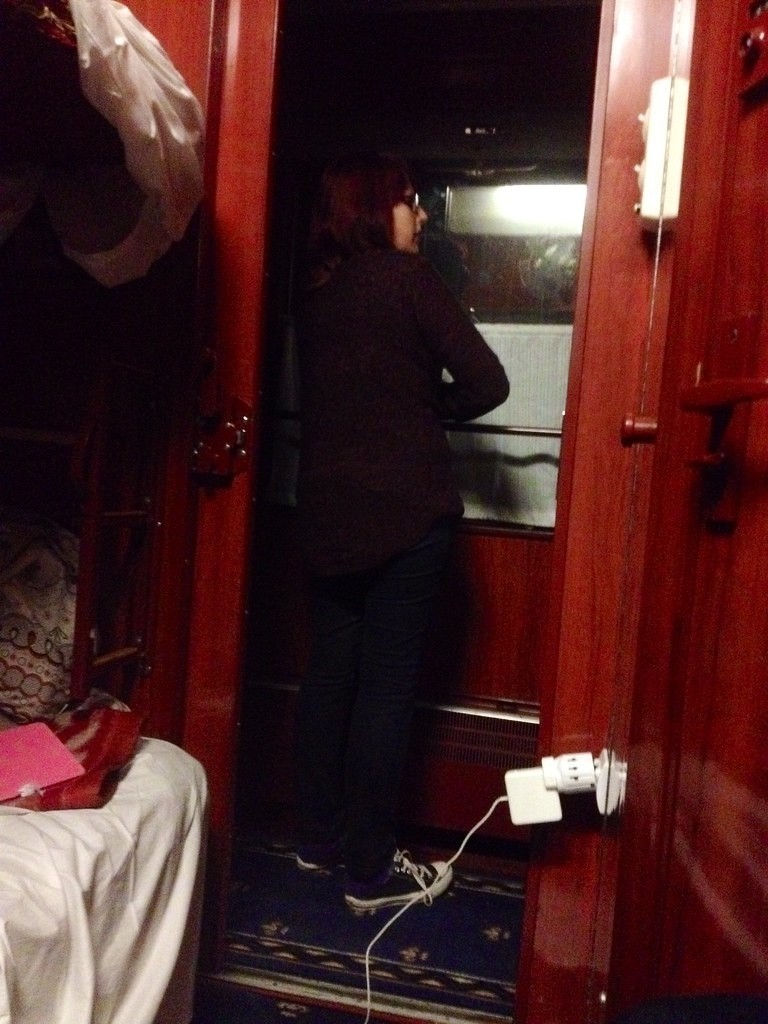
[0,0,230,1024]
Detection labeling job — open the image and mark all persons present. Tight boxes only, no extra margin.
[295,140,510,912]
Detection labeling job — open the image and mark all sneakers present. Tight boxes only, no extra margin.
[297,852,330,870]
[345,848,453,912]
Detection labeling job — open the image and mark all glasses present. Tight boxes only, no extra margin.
[399,191,421,214]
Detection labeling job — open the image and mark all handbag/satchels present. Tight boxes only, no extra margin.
[0,511,101,722]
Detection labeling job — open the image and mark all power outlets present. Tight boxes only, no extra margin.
[595,749,627,814]
